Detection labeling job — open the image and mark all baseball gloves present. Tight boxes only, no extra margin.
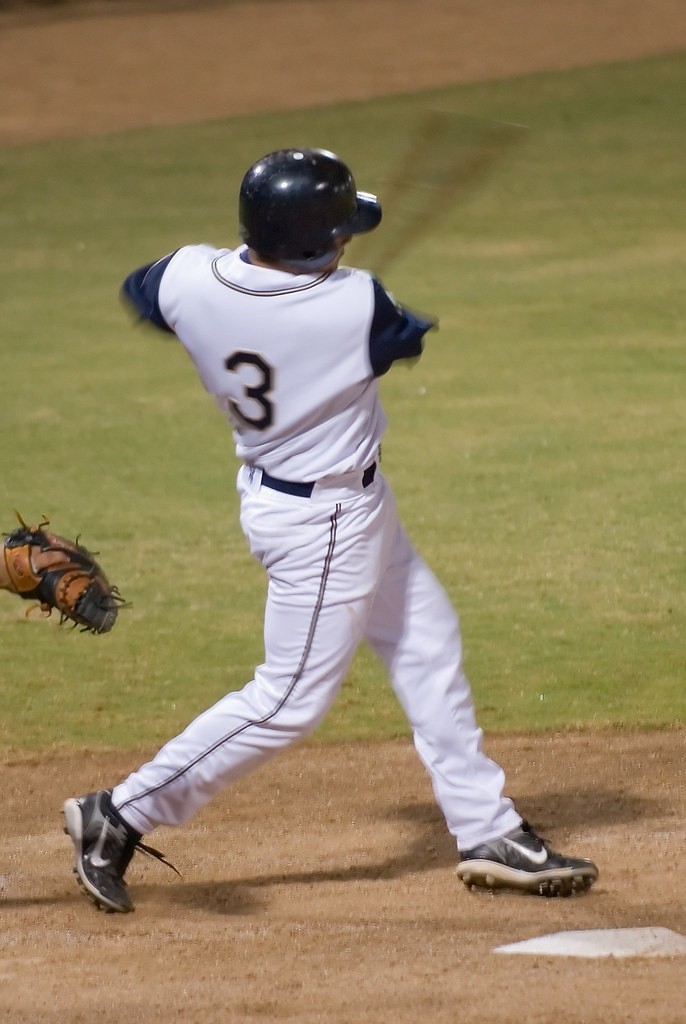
[0,524,119,637]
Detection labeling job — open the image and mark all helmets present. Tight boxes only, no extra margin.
[238,148,382,271]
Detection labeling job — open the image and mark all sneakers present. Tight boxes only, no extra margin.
[58,788,182,914]
[455,820,599,898]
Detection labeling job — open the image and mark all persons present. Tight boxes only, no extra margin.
[62,143,599,912]
[1,524,119,633]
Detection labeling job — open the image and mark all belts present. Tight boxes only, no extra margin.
[261,461,377,498]
